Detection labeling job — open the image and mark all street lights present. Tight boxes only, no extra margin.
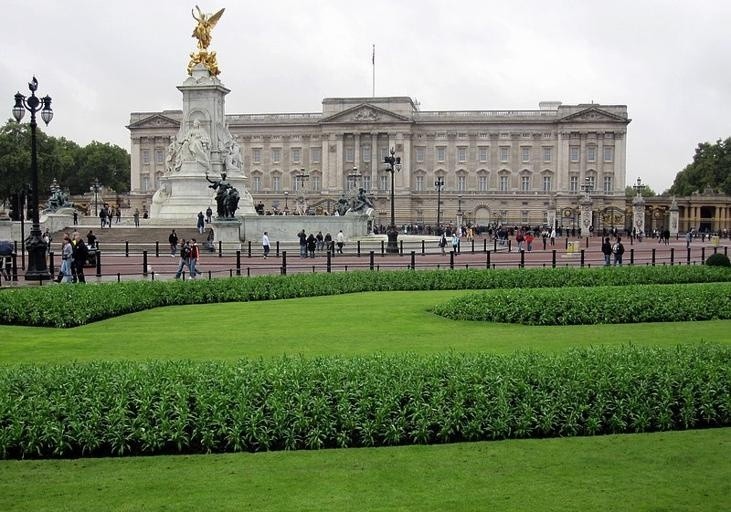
[633,177,646,232]
[348,166,363,213]
[581,178,594,247]
[385,146,403,256]
[435,176,445,226]
[12,74,54,282]
[284,192,290,215]
[296,168,310,215]
[91,177,102,217]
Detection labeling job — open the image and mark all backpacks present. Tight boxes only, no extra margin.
[612,243,620,254]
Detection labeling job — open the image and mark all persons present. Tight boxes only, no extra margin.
[589,223,730,245]
[298,229,345,255]
[43,229,97,283]
[73,203,149,228]
[373,220,480,239]
[339,194,349,206]
[256,201,348,216]
[209,172,232,218]
[168,207,213,279]
[263,232,271,258]
[191,8,213,50]
[354,187,374,211]
[600,237,623,265]
[439,233,459,255]
[489,223,583,251]
